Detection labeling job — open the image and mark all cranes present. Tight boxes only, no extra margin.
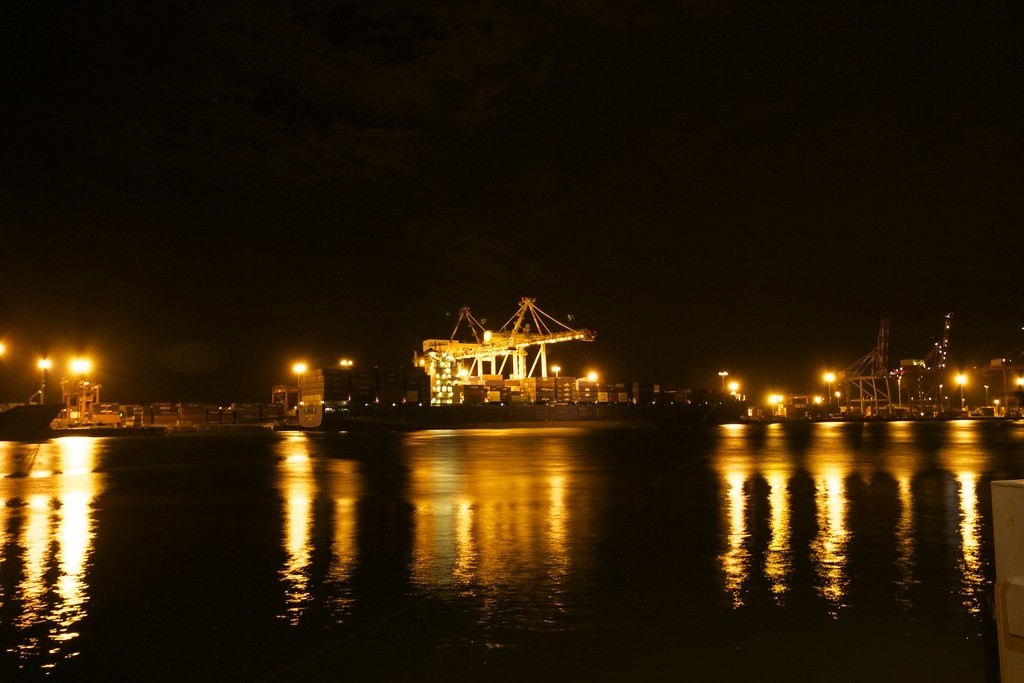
[413,294,597,405]
[839,311,953,414]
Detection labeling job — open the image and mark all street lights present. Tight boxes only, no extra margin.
[719,371,728,393]
[956,374,967,409]
[826,372,834,404]
